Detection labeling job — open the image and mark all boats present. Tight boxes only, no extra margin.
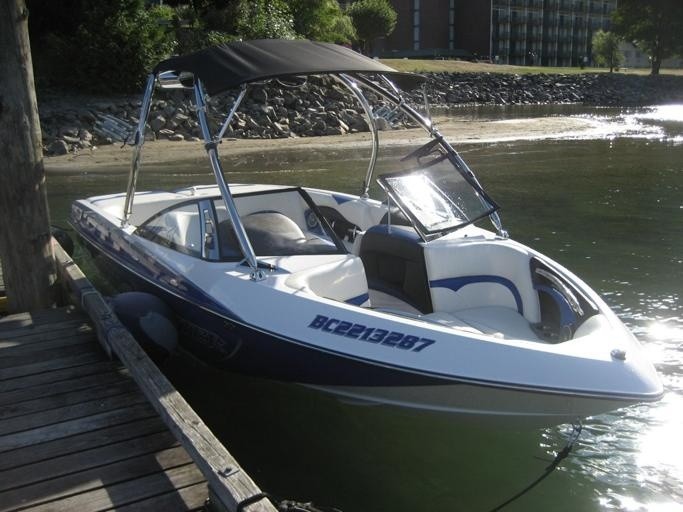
[68,37,667,421]
